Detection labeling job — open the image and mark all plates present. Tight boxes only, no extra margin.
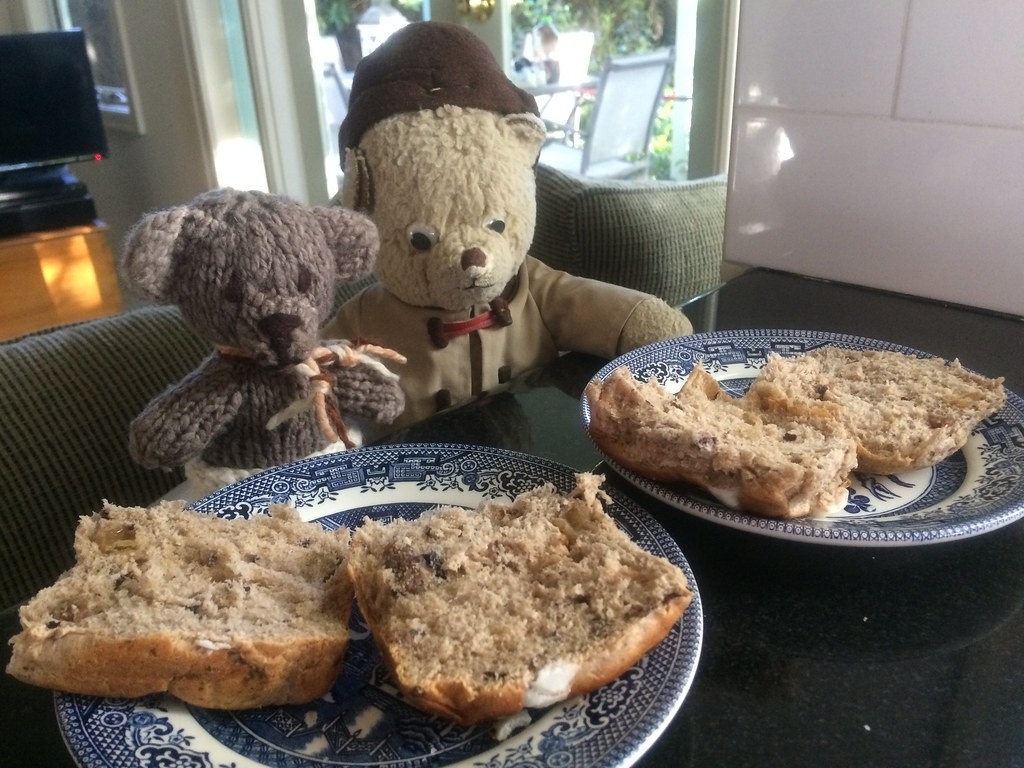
[54,444,703,767]
[580,329,1024,546]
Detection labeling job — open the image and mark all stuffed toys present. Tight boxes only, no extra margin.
[316,20,695,445]
[119,187,406,503]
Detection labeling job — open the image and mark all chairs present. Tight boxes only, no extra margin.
[536,47,679,183]
[538,30,595,147]
[323,63,354,155]
[0,302,220,768]
[526,162,731,308]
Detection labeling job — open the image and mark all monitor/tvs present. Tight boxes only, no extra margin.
[0,28,109,207]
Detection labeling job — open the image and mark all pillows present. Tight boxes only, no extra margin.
[562,172,730,307]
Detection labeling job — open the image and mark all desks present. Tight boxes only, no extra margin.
[0,255,1024,768]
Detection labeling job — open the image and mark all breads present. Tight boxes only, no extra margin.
[344,471,693,741]
[584,362,859,519]
[749,347,1008,474]
[3,499,353,709]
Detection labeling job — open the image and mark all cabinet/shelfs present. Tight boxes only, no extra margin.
[0,222,129,340]
